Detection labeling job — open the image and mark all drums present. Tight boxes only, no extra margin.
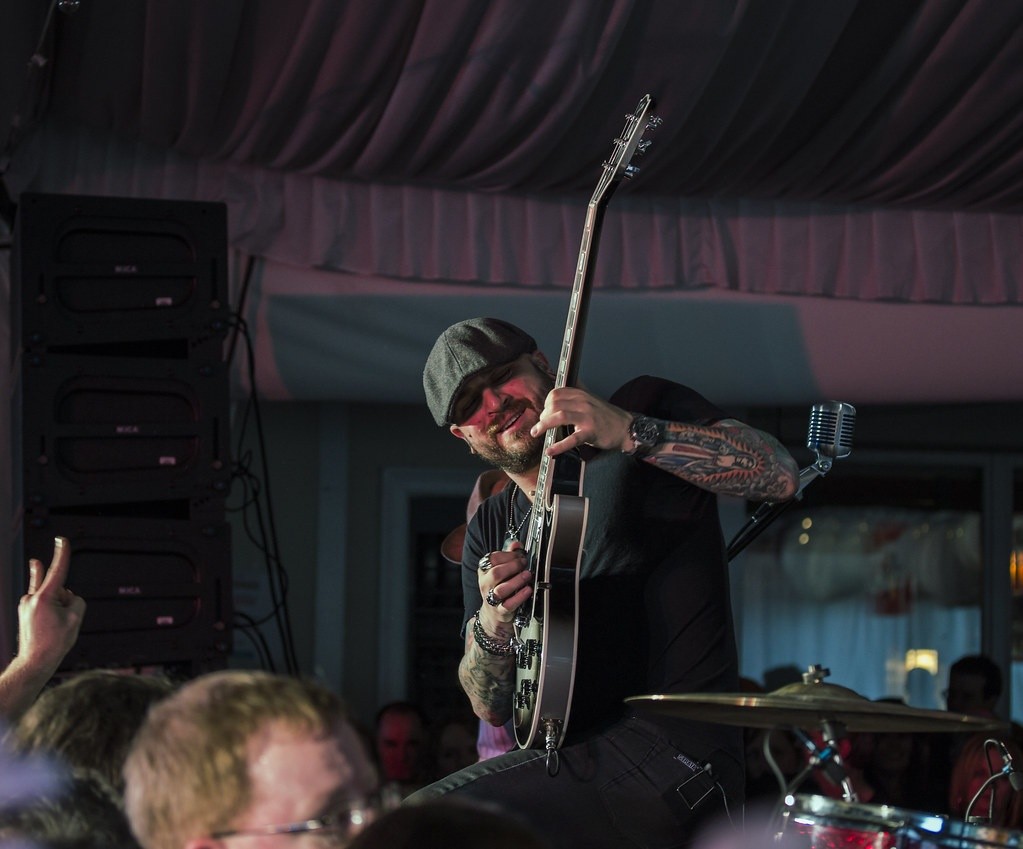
[771,794,1021,849]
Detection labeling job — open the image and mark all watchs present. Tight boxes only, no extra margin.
[621,412,659,458]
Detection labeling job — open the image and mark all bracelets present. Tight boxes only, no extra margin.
[473,611,515,656]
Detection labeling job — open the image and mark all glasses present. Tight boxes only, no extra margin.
[946,689,996,704]
[202,778,402,849]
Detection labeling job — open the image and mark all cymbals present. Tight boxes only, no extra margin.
[618,678,1001,735]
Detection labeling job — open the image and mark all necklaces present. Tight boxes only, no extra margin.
[503,485,531,552]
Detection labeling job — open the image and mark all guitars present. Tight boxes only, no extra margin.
[510,91,665,753]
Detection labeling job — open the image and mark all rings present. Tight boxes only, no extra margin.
[486,590,502,606]
[478,553,494,572]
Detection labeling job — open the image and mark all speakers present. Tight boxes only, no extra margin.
[13,191,231,672]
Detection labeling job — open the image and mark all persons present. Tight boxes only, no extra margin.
[0,535,170,849]
[126,672,379,849]
[402,317,799,849]
[347,800,538,848]
[373,701,477,797]
[745,653,1023,830]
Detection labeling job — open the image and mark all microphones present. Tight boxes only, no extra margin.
[998,743,1023,791]
[806,399,857,458]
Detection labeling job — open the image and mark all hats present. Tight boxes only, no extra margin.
[425,317,539,426]
